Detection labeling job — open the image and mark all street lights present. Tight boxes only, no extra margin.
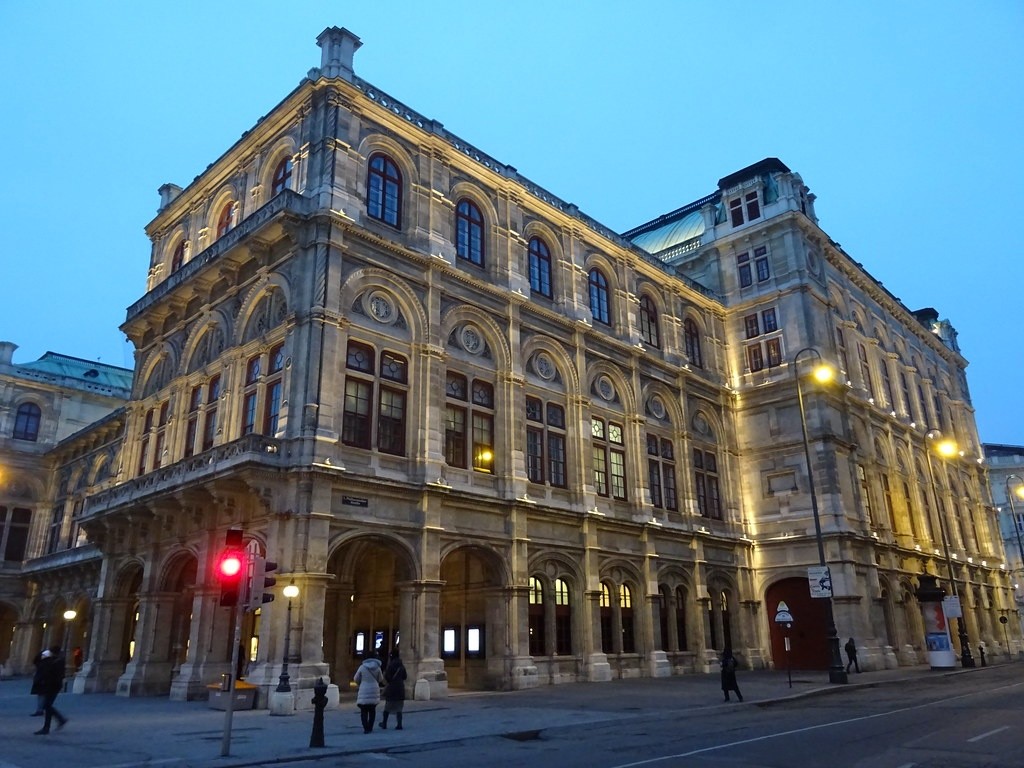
[922,427,977,669]
[62,610,78,656]
[270,576,299,716]
[794,347,849,683]
[1005,474,1024,568]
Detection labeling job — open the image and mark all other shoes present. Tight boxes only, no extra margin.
[59,719,68,726]
[395,726,403,729]
[29,711,44,716]
[33,728,50,735]
[378,722,387,729]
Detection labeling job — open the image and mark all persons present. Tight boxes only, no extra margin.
[378,648,408,730]
[353,652,384,734]
[29,650,53,717]
[73,647,82,669]
[236,639,245,682]
[845,638,862,674]
[719,645,744,702]
[34,645,67,736]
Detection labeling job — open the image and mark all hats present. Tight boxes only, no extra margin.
[42,650,51,657]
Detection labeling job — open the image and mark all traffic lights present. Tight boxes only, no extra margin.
[248,556,277,613]
[216,529,244,608]
